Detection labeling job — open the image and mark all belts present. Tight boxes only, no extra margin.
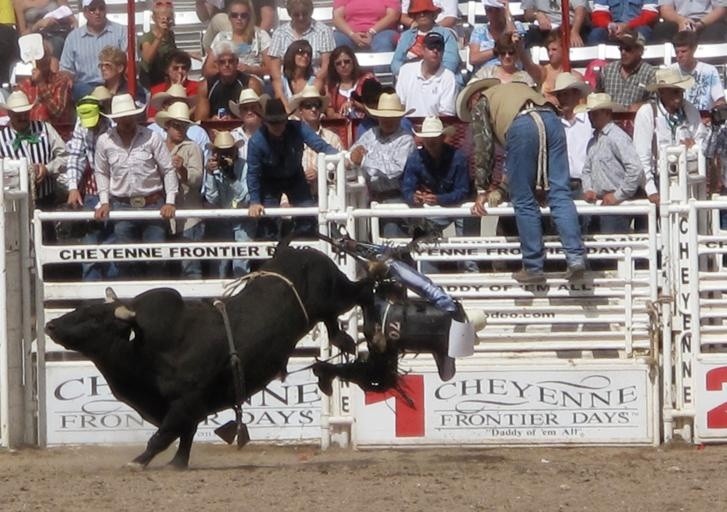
[114,192,162,208]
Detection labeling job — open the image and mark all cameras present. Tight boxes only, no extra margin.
[217,154,233,177]
[515,31,528,41]
[166,16,175,27]
[712,104,727,126]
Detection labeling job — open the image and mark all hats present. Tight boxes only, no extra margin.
[351,79,416,118]
[574,93,624,113]
[456,300,486,344]
[648,69,694,89]
[455,78,501,122]
[228,86,331,122]
[205,132,245,151]
[547,72,591,98]
[411,117,455,138]
[619,31,645,46]
[424,33,444,45]
[77,86,200,127]
[408,0,441,14]
[0,91,38,113]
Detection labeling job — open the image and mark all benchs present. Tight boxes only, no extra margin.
[64,0,727,126]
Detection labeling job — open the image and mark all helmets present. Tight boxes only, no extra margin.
[500,52,515,55]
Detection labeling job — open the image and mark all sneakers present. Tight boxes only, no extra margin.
[513,271,545,282]
[569,270,593,283]
[313,361,335,395]
[331,221,347,252]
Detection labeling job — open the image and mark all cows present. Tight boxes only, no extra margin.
[42,229,389,474]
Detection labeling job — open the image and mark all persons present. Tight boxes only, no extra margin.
[1,1,727,283]
[309,230,488,397]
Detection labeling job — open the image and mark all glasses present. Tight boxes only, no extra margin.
[427,42,443,50]
[89,2,308,18]
[297,48,312,56]
[98,60,235,70]
[336,60,350,65]
[619,47,632,51]
